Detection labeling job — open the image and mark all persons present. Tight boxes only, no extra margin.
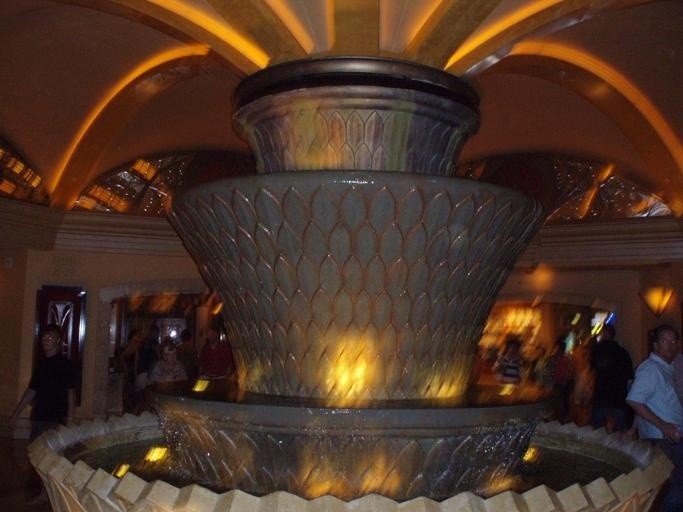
[491,324,636,441]
[619,324,683,512]
[7,323,76,505]
[121,323,237,417]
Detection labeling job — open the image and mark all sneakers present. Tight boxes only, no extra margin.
[24,494,49,506]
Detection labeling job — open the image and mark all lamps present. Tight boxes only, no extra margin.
[637,284,676,318]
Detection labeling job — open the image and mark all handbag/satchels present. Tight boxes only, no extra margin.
[134,371,149,391]
